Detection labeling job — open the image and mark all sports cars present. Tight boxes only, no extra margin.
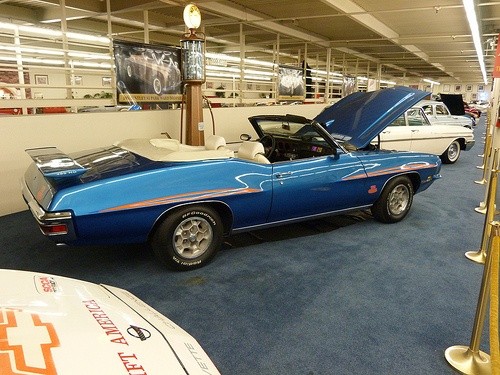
[19,84,446,271]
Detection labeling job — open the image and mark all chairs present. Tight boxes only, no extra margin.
[237,142,270,165]
[205,135,226,151]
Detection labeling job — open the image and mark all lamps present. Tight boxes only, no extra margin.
[182,4,203,40]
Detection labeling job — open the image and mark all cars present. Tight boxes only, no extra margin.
[397,100,490,132]
[369,105,476,165]
[0,267,221,375]
